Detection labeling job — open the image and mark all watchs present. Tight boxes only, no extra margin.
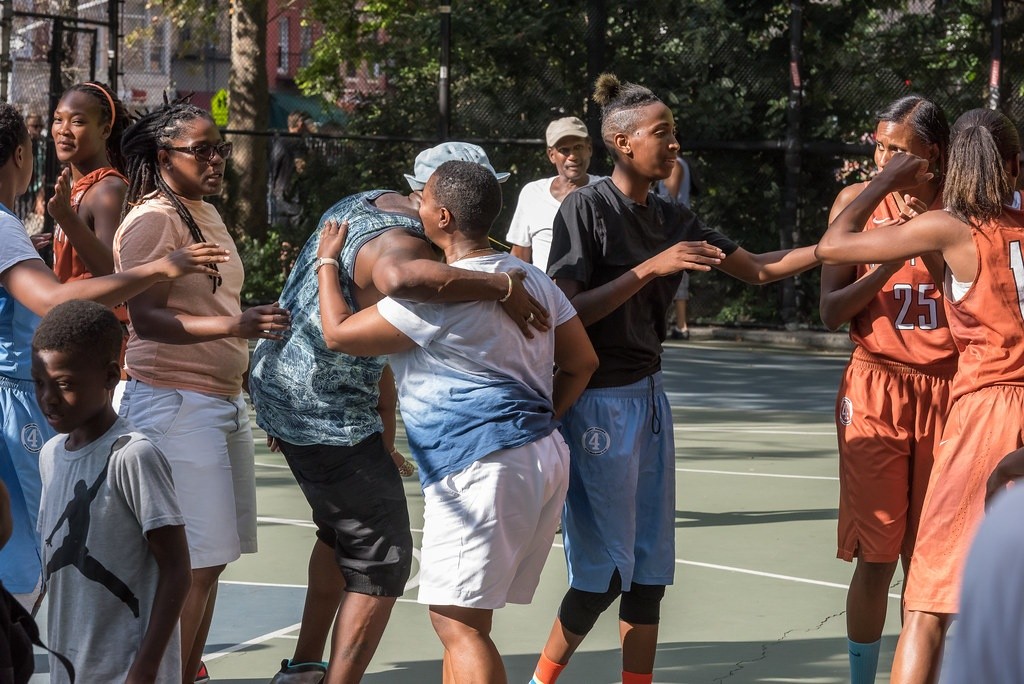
[313,258,340,274]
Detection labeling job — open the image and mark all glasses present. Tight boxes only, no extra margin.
[27,124,45,129]
[161,140,233,164]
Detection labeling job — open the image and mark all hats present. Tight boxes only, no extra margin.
[546,117,589,148]
[404,141,512,192]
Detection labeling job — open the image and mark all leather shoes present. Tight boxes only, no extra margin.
[391,447,415,477]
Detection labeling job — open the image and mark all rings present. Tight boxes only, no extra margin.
[526,313,535,322]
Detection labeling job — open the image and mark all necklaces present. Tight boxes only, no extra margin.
[457,247,493,261]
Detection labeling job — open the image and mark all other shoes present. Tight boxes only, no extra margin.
[672,325,690,339]
[194,660,210,684]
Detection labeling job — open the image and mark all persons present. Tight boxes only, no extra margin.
[814,93,1024,684]
[524,72,928,684]
[248,141,599,684]
[506,117,689,533]
[0,80,293,683]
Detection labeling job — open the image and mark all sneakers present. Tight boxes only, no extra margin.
[270,659,328,684]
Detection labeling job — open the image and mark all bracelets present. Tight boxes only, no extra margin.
[499,271,513,303]
